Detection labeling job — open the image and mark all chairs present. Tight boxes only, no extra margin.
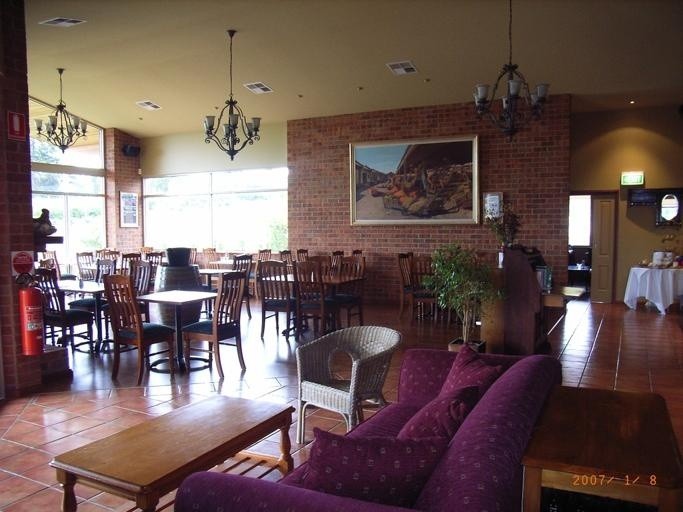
[295,325,404,444]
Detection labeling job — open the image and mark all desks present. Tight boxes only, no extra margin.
[623,266,683,316]
[543,294,566,337]
[568,264,590,301]
[519,384,683,512]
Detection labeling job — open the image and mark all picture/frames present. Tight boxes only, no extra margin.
[348,133,480,226]
[120,190,140,228]
[483,192,503,226]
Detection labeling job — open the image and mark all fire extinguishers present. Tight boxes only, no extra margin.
[15,272,45,356]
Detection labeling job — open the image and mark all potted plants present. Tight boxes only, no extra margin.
[422,245,511,356]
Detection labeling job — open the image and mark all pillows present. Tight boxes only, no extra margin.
[298,428,449,509]
[436,343,504,402]
[396,386,479,442]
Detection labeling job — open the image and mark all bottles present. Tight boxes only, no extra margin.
[497,246,504,269]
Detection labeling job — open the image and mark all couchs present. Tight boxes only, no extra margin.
[175,349,562,512]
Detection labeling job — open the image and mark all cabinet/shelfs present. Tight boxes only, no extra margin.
[627,188,683,226]
[482,245,553,355]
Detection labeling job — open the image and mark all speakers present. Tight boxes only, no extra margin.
[127,146,140,156]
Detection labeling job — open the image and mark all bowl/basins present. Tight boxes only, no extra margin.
[638,262,671,269]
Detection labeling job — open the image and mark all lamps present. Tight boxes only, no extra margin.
[32,68,87,155]
[203,29,261,161]
[473,0,550,142]
